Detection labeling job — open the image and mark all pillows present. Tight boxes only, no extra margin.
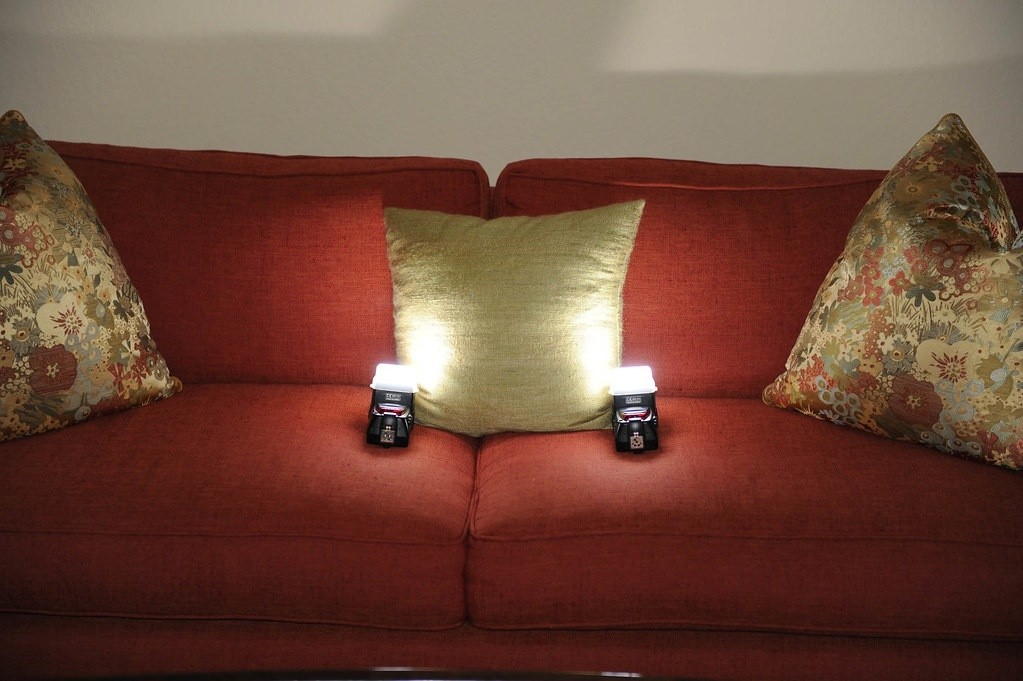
[385,199,645,437]
[762,113,1022,470]
[0,110,182,442]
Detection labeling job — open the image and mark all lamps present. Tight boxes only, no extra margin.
[365,363,420,447]
[607,366,658,454]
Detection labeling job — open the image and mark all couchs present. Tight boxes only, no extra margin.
[0,140,1022,681]
